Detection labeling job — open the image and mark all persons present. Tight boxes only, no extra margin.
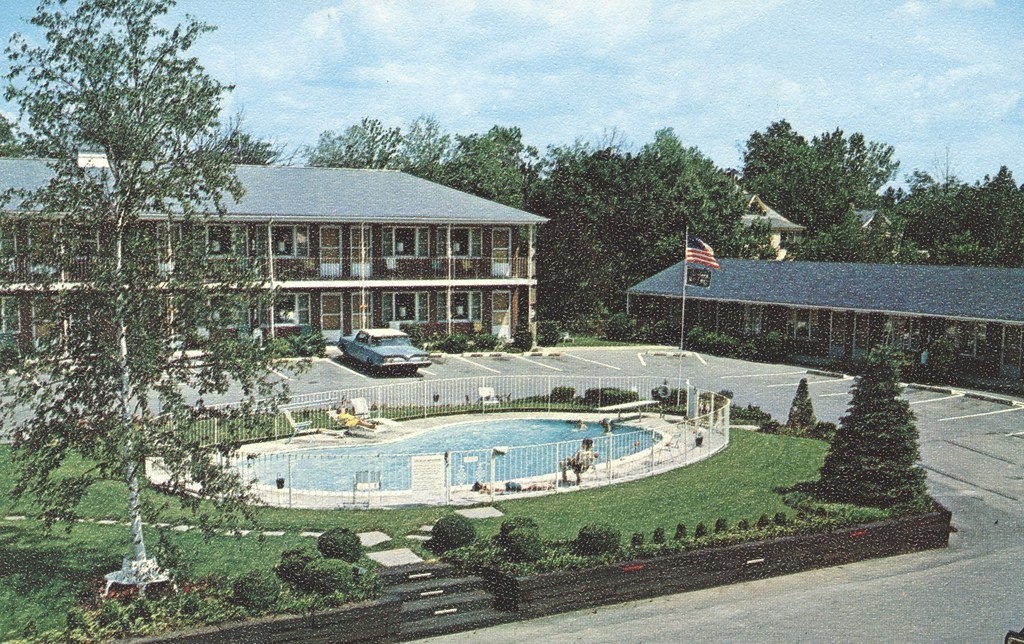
[559,418,613,483]
[336,406,380,429]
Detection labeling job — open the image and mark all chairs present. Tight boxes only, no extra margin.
[352,471,382,506]
[658,435,684,463]
[326,397,401,437]
[479,387,500,414]
[285,410,316,444]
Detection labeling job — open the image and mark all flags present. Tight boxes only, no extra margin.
[686,265,712,289]
[688,234,720,271]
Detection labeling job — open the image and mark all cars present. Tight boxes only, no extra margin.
[337,328,432,375]
